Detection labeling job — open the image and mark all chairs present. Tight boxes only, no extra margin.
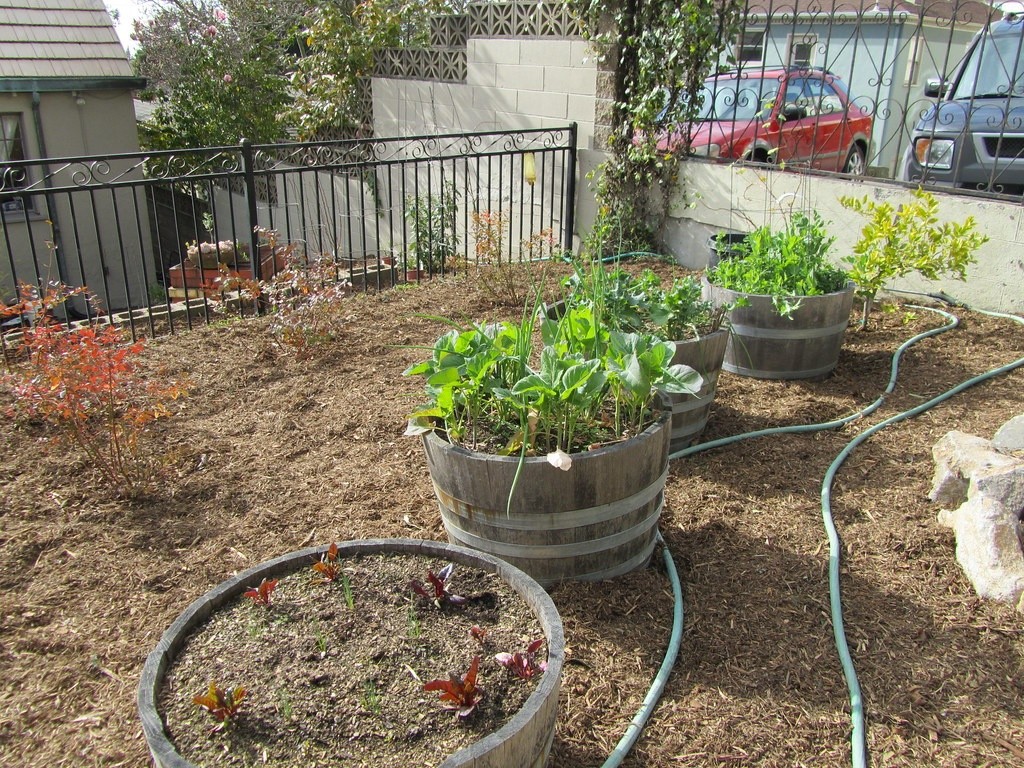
[786,93,798,103]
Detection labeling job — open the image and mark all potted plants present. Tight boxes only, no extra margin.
[701,210,856,379]
[406,256,424,280]
[540,215,753,454]
[137,538,565,768]
[389,254,703,585]
[187,212,234,269]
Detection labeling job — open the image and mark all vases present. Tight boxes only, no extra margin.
[382,257,397,266]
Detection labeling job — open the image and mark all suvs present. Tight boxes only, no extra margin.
[632,65,872,182]
[898,12,1024,196]
